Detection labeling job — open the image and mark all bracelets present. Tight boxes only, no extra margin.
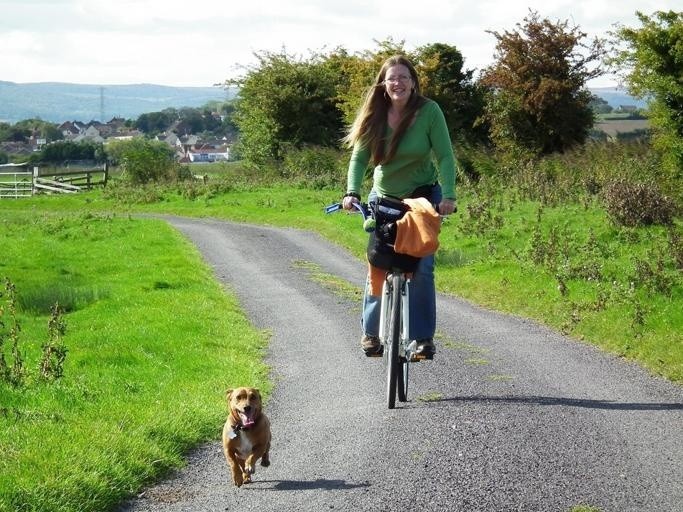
[343,192,361,201]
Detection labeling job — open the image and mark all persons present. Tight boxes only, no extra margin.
[343,56,456,353]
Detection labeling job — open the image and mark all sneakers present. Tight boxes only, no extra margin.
[361,334,381,353]
[416,338,436,356]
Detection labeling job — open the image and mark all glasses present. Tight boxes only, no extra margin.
[385,73,413,83]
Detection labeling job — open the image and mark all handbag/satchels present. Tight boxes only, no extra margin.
[366,197,421,273]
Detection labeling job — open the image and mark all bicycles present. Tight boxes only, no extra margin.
[325,201,458,410]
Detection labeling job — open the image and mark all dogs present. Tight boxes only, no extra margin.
[221,387,272,488]
